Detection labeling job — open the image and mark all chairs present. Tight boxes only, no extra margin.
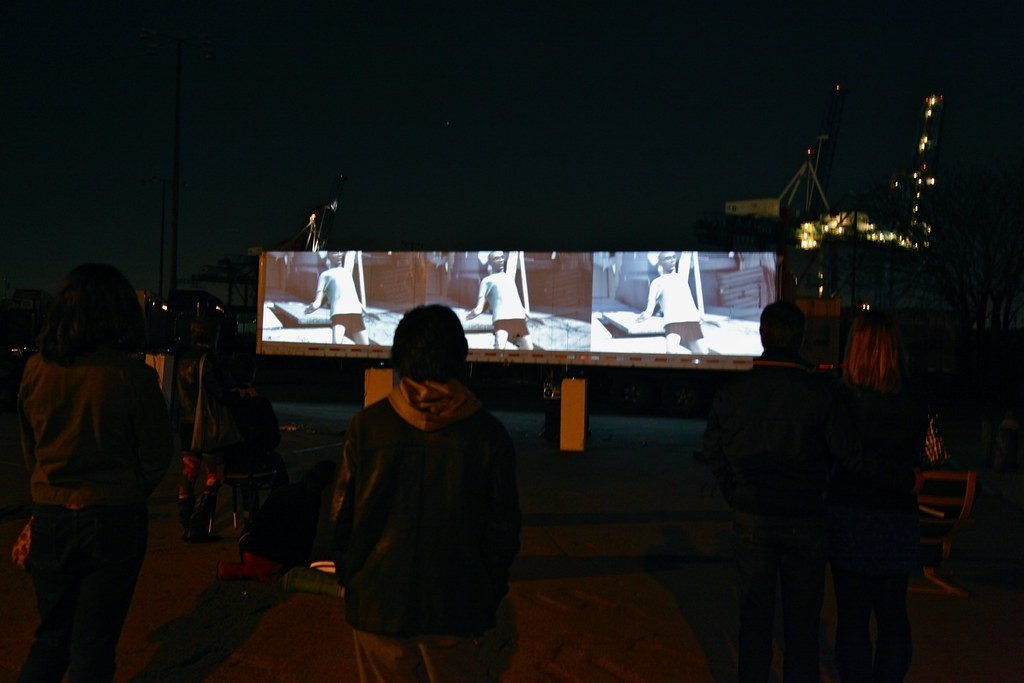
[906,468,977,597]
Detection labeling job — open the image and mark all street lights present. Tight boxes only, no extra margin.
[139,27,217,298]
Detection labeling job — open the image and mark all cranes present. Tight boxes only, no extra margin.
[804,83,847,223]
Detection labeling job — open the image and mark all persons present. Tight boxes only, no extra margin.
[14,262,173,683]
[829,303,929,683]
[306,251,371,346]
[327,305,520,683]
[175,317,230,544]
[466,251,538,349]
[637,251,708,354]
[705,298,925,683]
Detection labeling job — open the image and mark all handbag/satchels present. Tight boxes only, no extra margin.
[190,352,245,452]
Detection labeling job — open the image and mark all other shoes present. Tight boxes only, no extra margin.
[182,529,221,543]
